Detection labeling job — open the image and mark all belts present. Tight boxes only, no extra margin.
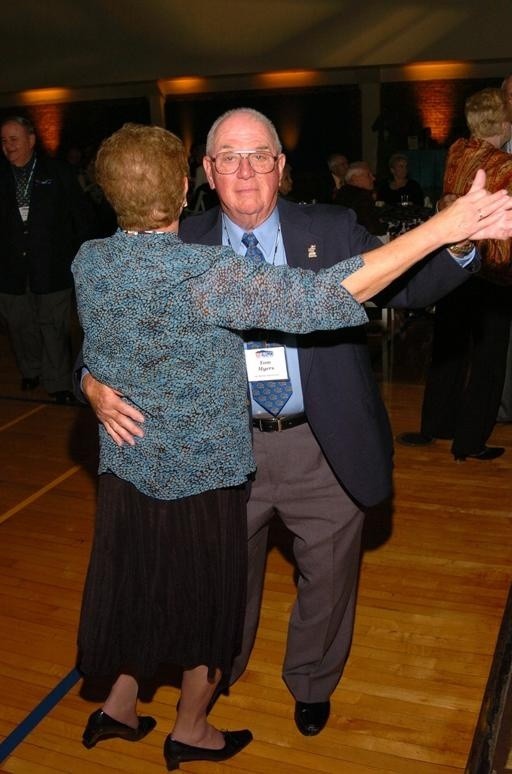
[250,412,308,432]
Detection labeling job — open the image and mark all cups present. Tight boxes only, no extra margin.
[399,194,408,206]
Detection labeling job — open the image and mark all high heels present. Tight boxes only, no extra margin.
[81,707,158,746]
[162,729,254,774]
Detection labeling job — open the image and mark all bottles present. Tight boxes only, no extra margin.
[424,196,438,209]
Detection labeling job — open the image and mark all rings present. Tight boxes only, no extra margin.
[478,208,483,219]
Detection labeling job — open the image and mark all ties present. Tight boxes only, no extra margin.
[240,232,293,419]
[15,167,33,210]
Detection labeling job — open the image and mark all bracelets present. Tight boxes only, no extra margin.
[448,242,475,254]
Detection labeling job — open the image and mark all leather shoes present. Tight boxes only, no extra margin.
[293,698,331,738]
[450,445,506,462]
[175,695,216,720]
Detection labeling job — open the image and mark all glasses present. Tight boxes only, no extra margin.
[209,150,278,175]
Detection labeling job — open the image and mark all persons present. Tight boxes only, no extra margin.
[0,116,97,404]
[420,87,512,464]
[435,74,512,424]
